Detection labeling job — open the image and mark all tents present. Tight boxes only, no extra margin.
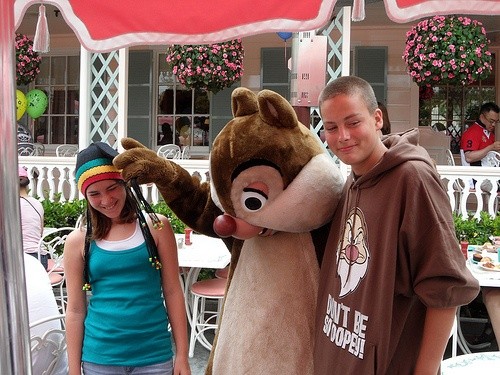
[0,0,500,375]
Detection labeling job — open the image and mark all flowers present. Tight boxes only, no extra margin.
[166,39,247,89]
[402,15,492,89]
[14,31,42,85]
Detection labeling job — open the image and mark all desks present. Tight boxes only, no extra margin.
[441,244,500,375]
[173,232,231,352]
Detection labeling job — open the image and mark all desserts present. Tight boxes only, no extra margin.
[473,253,482,262]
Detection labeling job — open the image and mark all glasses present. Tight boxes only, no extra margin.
[483,113,500,126]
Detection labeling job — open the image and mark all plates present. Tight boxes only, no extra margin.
[469,255,493,263]
[478,262,500,271]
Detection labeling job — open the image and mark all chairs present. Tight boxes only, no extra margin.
[25,144,229,375]
[445,149,500,214]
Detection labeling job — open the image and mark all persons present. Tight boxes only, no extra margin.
[378,101,391,149]
[63,142,192,375]
[315,76,480,375]
[460,102,500,193]
[18,167,48,270]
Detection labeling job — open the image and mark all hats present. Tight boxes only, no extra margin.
[19,166,27,176]
[75,142,128,198]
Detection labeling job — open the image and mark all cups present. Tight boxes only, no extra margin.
[184,227,192,245]
[460,242,468,260]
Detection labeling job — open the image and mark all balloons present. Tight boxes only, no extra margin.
[25,90,48,119]
[16,89,27,121]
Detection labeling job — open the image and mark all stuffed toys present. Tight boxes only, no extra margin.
[112,87,344,375]
